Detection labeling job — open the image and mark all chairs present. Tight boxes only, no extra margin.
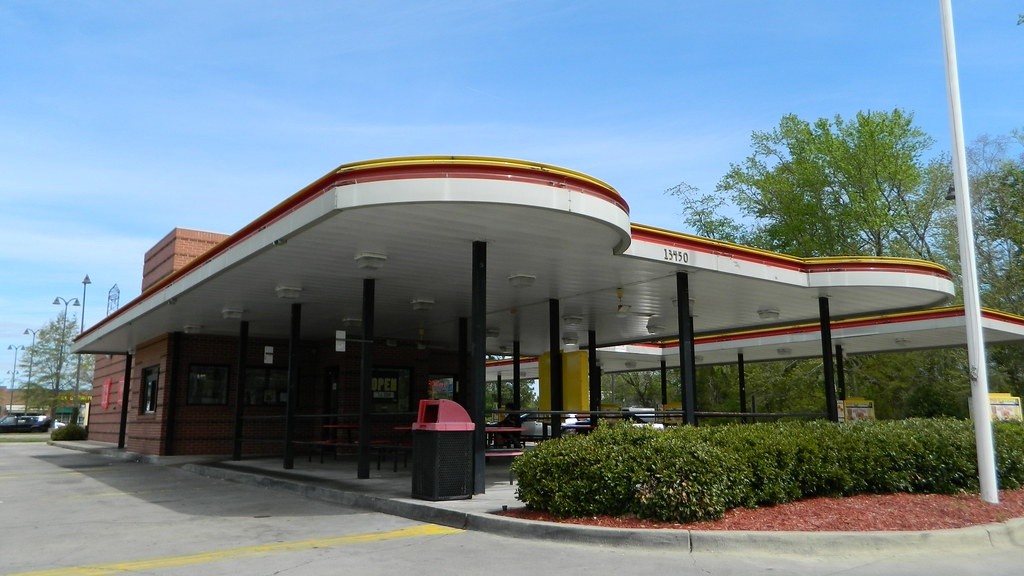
[288,407,603,458]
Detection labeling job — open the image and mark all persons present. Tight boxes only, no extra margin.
[490,403,516,450]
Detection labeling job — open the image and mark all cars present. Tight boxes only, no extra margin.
[0,412,65,429]
[485,404,664,439]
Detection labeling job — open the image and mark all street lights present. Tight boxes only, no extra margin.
[6,343,26,412]
[24,326,44,416]
[73,275,91,411]
[52,295,80,414]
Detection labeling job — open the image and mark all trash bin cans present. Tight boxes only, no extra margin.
[410,398,477,501]
